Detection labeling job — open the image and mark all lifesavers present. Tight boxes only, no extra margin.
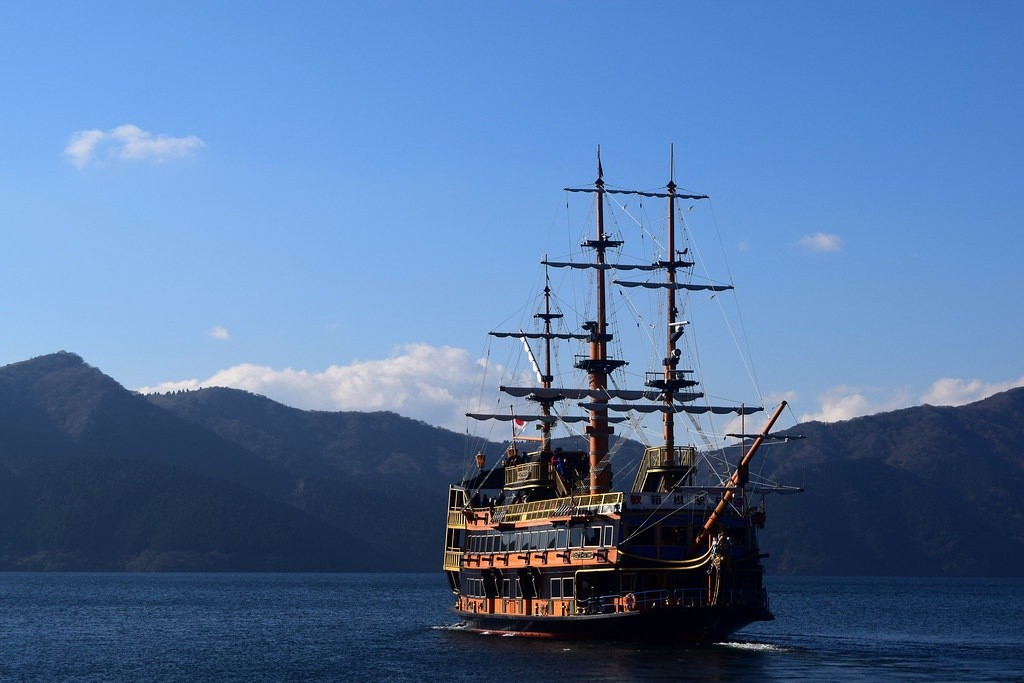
[625,592,636,610]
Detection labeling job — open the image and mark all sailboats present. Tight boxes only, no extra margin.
[445,141,809,644]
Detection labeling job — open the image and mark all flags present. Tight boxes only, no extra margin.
[514,418,528,431]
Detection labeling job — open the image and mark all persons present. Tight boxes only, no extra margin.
[551,451,564,475]
